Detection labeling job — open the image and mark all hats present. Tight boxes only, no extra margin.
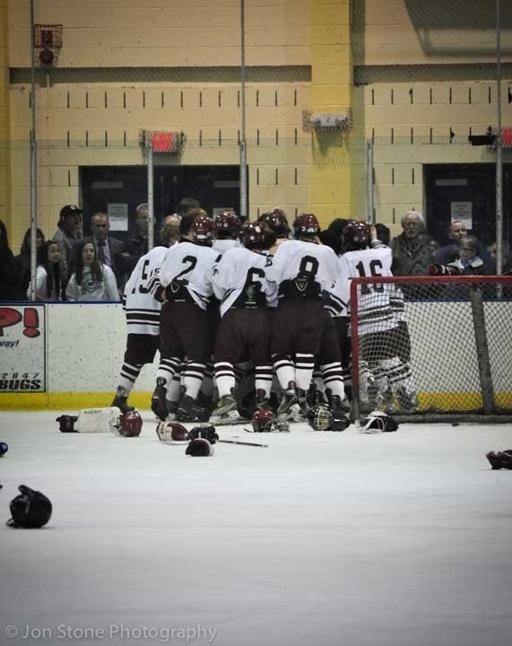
[60,205,85,218]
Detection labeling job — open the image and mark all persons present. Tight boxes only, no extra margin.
[0,197,512,423]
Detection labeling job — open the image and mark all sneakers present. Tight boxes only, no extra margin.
[112,396,135,413]
[154,377,420,421]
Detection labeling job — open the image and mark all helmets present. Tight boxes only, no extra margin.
[250,410,290,434]
[340,221,373,250]
[190,208,319,247]
[112,411,143,438]
[306,402,351,432]
